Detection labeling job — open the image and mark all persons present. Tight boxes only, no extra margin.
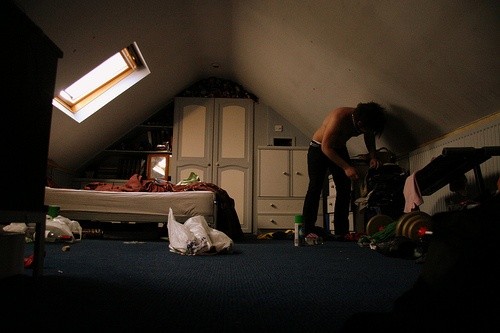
[301,102,391,243]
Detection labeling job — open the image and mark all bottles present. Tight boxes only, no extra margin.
[294,215,305,247]
[32,202,105,242]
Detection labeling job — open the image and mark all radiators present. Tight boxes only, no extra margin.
[407,116,500,219]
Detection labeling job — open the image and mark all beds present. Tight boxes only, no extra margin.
[45,161,218,232]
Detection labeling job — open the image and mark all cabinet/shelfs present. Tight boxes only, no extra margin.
[169,96,254,233]
[254,146,324,238]
[1,1,64,275]
[325,173,355,234]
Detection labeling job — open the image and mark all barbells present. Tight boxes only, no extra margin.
[365,210,440,263]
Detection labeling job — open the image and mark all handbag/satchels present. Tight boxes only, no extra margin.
[4,216,83,243]
[167,207,235,256]
